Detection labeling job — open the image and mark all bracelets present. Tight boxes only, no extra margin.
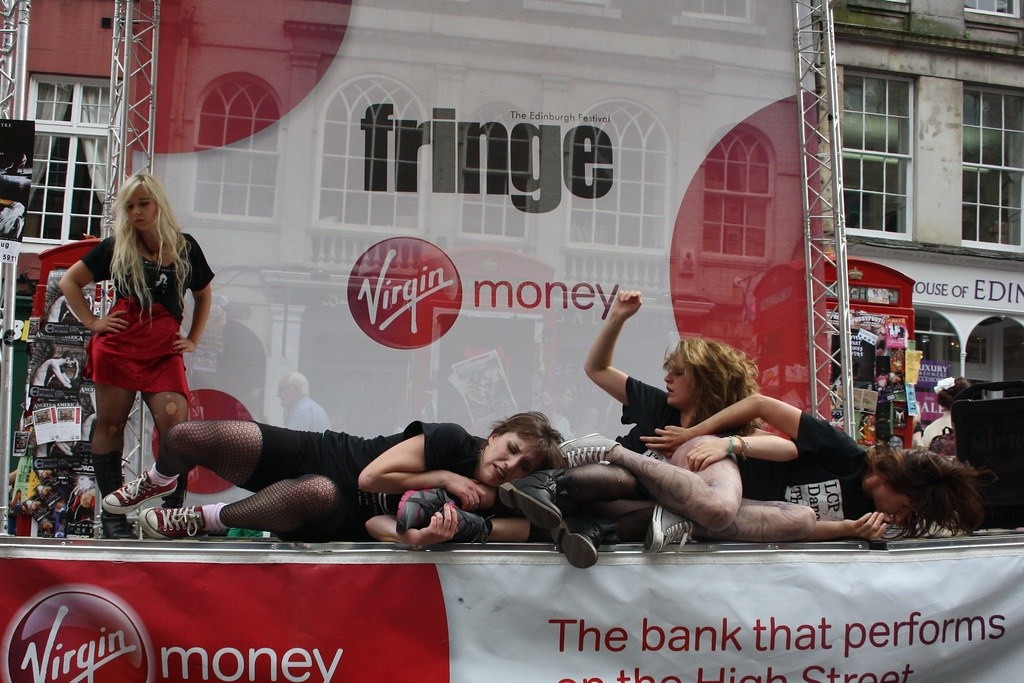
[729,435,746,457]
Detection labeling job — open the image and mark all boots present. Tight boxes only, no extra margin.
[89,450,138,540]
[161,476,187,509]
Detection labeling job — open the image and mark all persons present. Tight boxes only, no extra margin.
[16,405,95,459]
[12,470,101,531]
[877,350,906,449]
[59,173,215,539]
[100,290,979,569]
[48,286,94,326]
[32,352,79,389]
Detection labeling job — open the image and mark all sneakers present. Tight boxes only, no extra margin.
[102,466,178,515]
[643,503,699,553]
[396,487,455,537]
[138,503,228,541]
[557,432,622,469]
[439,500,495,545]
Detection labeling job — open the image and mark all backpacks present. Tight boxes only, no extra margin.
[929,425,957,461]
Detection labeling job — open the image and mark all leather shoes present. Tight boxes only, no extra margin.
[498,467,569,530]
[553,508,619,569]
